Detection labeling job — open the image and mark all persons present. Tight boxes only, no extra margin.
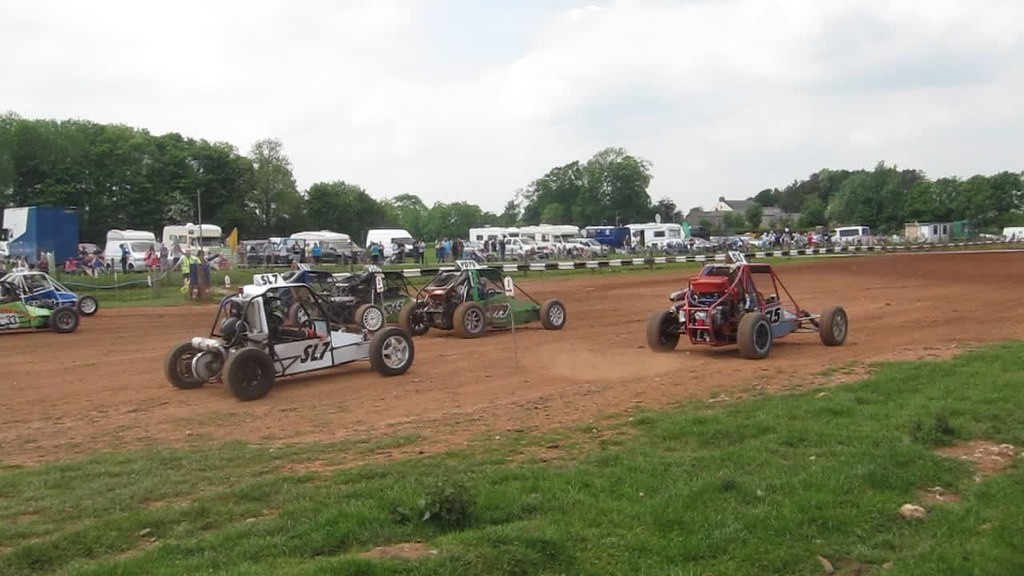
[234,234,508,268]
[737,227,837,253]
[0,229,210,299]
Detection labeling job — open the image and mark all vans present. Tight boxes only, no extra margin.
[162,222,223,263]
[366,229,418,259]
[469,221,764,256]
[104,229,162,274]
[827,226,870,245]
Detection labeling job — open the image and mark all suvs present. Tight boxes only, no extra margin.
[237,231,366,264]
[402,259,568,339]
[0,268,99,336]
[167,273,413,400]
[644,250,849,358]
[272,262,424,334]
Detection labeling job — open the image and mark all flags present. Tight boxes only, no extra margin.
[228,229,238,254]
